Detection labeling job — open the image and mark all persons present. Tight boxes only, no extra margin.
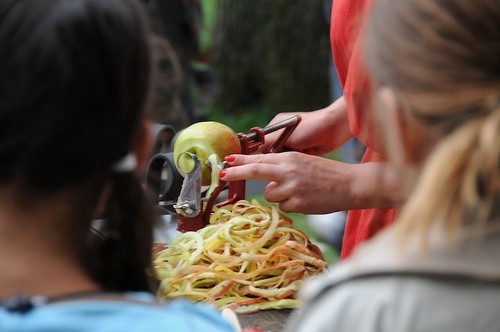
[220,0,500,332]
[0,0,239,332]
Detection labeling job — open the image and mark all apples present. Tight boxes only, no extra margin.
[173,120,242,186]
